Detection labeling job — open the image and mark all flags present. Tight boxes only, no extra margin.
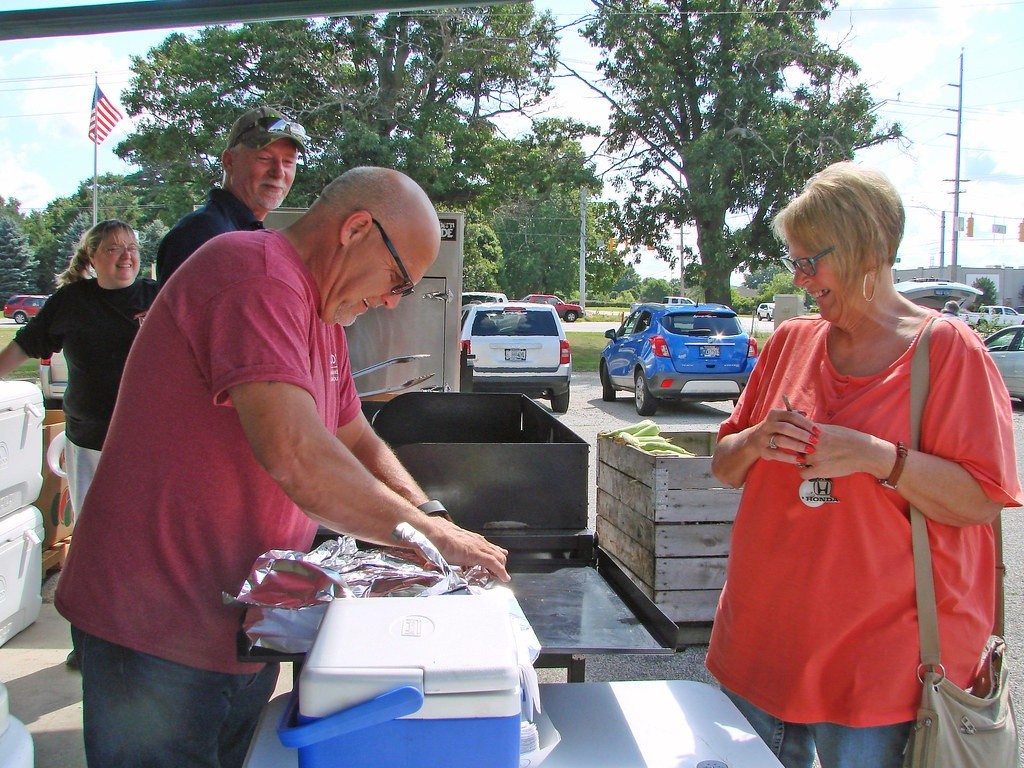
[88,84,122,146]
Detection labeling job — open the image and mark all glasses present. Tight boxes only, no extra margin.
[780,246,835,277]
[233,117,306,141]
[93,244,138,256]
[371,217,416,297]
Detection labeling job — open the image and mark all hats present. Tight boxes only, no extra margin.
[226,107,306,157]
[941,300,959,316]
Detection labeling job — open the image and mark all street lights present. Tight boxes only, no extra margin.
[910,196,946,279]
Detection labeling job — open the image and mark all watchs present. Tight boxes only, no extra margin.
[877,440,908,491]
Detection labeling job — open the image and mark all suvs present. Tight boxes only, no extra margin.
[462,290,509,307]
[460,299,572,413]
[3,293,50,325]
[597,302,760,416]
[521,292,584,322]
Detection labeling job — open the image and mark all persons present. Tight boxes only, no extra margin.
[940,300,960,317]
[157,106,310,293]
[0,219,157,528]
[706,160,1018,768]
[991,309,1001,315]
[49,164,512,766]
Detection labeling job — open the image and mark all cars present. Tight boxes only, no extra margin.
[893,275,983,313]
[38,347,70,400]
[756,302,775,321]
[983,324,1024,402]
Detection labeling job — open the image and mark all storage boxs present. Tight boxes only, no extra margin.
[0,382,75,649]
[595,430,747,653]
[276,597,522,768]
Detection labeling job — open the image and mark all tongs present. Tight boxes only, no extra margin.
[351,355,437,398]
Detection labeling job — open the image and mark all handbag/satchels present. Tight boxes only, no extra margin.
[902,642,1019,768]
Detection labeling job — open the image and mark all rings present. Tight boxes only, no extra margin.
[769,433,778,450]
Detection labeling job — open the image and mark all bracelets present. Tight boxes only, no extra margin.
[415,499,447,516]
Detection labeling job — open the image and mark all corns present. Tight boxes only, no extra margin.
[601,419,696,457]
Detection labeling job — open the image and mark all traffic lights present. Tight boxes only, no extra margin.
[606,238,618,253]
[1017,222,1024,242]
[965,217,975,238]
[646,232,657,250]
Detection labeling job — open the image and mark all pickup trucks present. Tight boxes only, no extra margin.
[958,306,1024,330]
[629,296,696,318]
